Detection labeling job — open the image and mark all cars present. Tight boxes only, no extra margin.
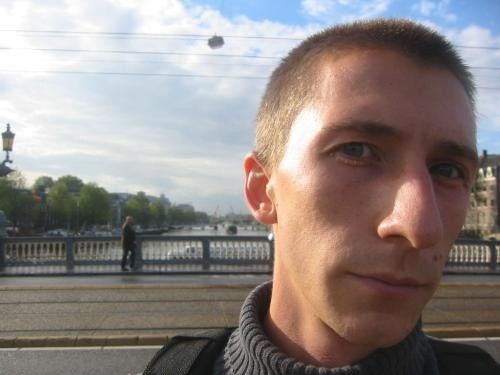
[165,245,214,259]
[8,222,185,236]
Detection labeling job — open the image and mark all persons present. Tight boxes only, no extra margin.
[120,214,139,271]
[137,16,500,375]
